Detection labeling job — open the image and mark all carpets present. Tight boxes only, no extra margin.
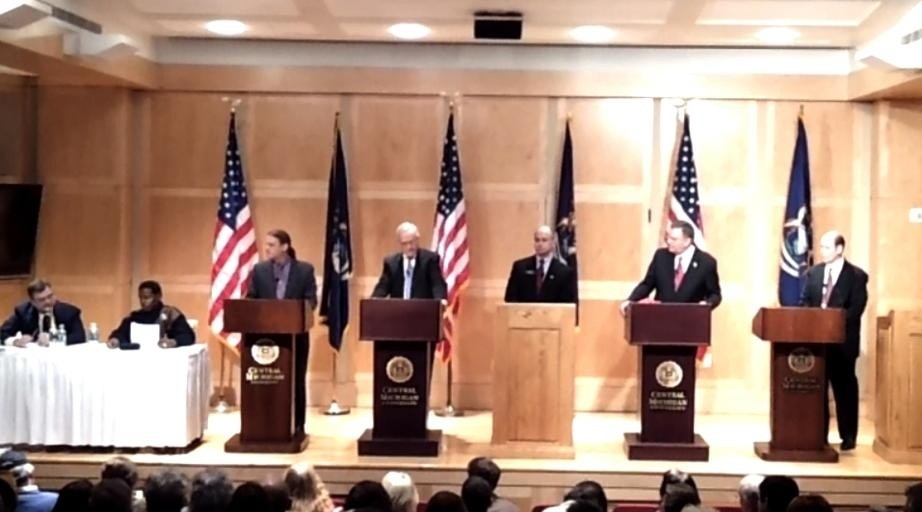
[535,259,546,296]
[43,315,51,332]
[406,259,413,280]
[820,269,833,311]
[674,256,685,291]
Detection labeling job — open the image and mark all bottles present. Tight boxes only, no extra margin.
[10,462,34,481]
[0,450,27,470]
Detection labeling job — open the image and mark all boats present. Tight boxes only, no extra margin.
[473,11,522,40]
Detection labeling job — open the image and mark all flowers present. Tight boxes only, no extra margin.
[43,307,51,333]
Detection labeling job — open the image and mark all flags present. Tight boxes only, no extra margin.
[431,112,470,364]
[205,112,259,359]
[319,128,354,353]
[778,116,813,306]
[556,119,578,326]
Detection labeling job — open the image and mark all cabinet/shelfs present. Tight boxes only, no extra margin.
[88,322,98,342]
[49,324,67,346]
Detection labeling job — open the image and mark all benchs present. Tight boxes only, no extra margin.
[778,116,813,306]
[556,119,578,326]
[205,112,259,359]
[431,112,470,364]
[319,128,354,353]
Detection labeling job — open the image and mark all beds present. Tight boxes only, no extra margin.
[662,114,707,360]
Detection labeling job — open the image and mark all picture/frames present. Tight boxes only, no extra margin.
[0,334,211,452]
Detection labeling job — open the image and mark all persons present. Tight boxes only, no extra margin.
[618,221,721,318]
[370,222,448,396]
[503,224,578,303]
[245,229,317,434]
[905,483,922,512]
[106,280,194,349]
[0,448,834,512]
[0,280,86,347]
[797,231,868,452]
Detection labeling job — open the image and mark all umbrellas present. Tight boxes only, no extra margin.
[662,114,707,360]
